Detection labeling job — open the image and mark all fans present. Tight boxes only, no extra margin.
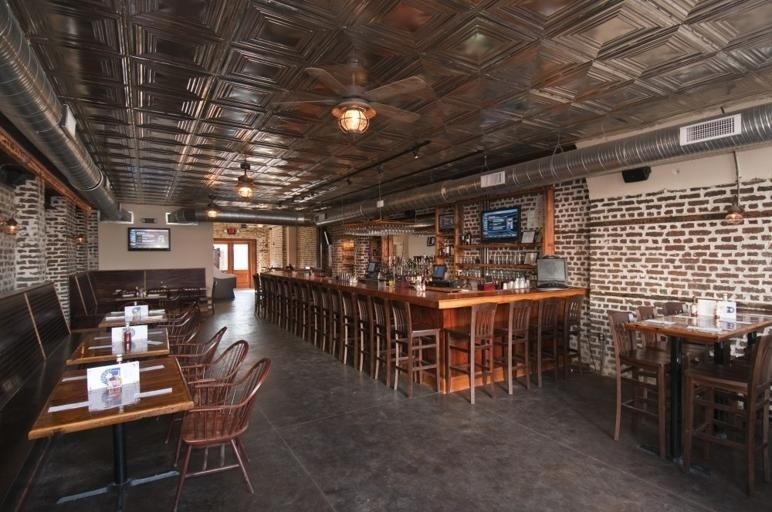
[270,43,433,121]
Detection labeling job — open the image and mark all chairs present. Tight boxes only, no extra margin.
[155,327,198,361]
[162,326,227,374]
[340,291,385,371]
[158,307,200,342]
[368,294,417,386]
[542,295,584,377]
[157,300,196,325]
[638,306,688,426]
[327,285,367,359]
[608,310,667,462]
[359,296,402,381]
[156,340,249,446]
[392,300,440,399]
[164,358,271,512]
[316,285,354,356]
[513,301,562,388]
[254,273,316,346]
[179,286,199,313]
[444,302,497,404]
[681,334,772,497]
[482,300,530,394]
[665,302,709,358]
[147,287,167,294]
[199,282,217,315]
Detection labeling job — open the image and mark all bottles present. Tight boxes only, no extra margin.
[396,256,435,277]
[485,267,537,289]
[124,324,131,352]
[462,252,480,264]
[488,247,526,265]
[461,266,481,277]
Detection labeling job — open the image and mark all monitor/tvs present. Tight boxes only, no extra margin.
[432,265,444,277]
[128,228,171,250]
[482,206,522,242]
[367,263,376,271]
[538,258,566,290]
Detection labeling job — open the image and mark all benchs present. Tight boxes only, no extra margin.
[0,281,95,512]
[75,272,122,322]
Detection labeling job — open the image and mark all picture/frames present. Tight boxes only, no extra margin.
[521,230,536,244]
[524,252,539,265]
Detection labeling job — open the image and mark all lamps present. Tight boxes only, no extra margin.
[723,194,743,224]
[206,194,220,218]
[237,162,252,197]
[331,98,376,138]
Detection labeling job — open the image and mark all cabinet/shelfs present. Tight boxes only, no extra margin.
[432,183,556,289]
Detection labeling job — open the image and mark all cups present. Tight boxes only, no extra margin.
[503,278,529,290]
